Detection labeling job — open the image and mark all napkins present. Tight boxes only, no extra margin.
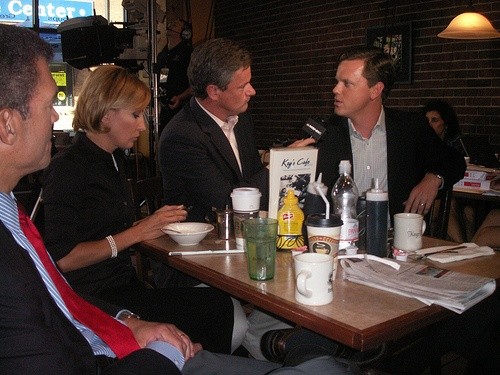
[416,242,494,263]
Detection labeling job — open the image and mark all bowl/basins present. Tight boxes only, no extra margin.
[160,221,215,247]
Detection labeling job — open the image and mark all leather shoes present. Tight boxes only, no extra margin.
[260,326,385,365]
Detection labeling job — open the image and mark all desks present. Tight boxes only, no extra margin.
[450,191,500,241]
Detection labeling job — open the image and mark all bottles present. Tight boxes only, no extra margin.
[277,189,305,249]
[331,159,359,256]
[303,172,332,216]
[215,207,234,240]
[366,177,389,259]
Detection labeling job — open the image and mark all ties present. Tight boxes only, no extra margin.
[18,206,142,360]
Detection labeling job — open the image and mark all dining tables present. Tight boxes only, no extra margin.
[132,235,500,353]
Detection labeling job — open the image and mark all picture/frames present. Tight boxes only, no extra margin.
[365,22,413,85]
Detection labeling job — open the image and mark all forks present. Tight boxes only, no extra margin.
[406,245,468,261]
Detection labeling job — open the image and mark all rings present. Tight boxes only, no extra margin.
[181,334,185,337]
[420,203,425,206]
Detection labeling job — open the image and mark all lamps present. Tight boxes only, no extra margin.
[437,0,500,39]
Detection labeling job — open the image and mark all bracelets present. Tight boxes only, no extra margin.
[106,234,117,258]
[119,311,138,321]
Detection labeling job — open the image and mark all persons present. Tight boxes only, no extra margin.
[423,102,500,248]
[156,18,193,138]
[315,45,466,237]
[0,24,385,375]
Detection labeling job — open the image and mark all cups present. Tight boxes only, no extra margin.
[259,210,268,224]
[294,253,334,306]
[357,196,366,250]
[305,212,344,281]
[242,218,279,281]
[394,212,426,251]
[230,186,262,250]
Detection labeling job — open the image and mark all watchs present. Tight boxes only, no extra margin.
[425,171,444,189]
